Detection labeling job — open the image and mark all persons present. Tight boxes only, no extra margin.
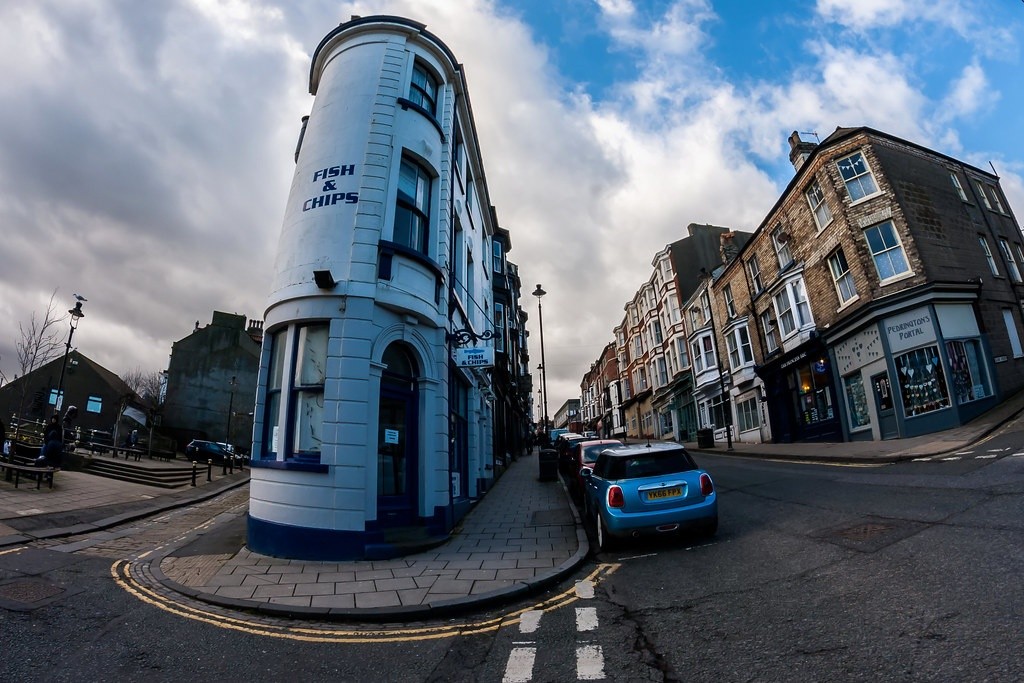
[44,415,62,447]
[119,429,139,456]
[523,431,534,456]
[31,431,62,480]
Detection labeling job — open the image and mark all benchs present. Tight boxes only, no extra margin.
[92,442,144,462]
[0,454,58,490]
[151,448,174,461]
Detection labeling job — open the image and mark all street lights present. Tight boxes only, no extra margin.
[43,301,85,419]
[698,267,735,451]
[222,375,238,468]
[149,369,169,442]
[536,364,547,427]
[536,389,545,422]
[532,283,550,441]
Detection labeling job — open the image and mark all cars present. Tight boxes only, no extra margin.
[579,440,718,552]
[185,439,243,467]
[565,440,626,499]
[545,427,594,477]
[216,441,234,454]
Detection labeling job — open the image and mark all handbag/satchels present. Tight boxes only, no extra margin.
[3,440,12,455]
[34,455,49,467]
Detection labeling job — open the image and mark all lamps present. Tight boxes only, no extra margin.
[313,270,337,289]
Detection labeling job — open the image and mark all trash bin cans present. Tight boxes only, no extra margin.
[538,446,558,482]
[697,428,715,449]
